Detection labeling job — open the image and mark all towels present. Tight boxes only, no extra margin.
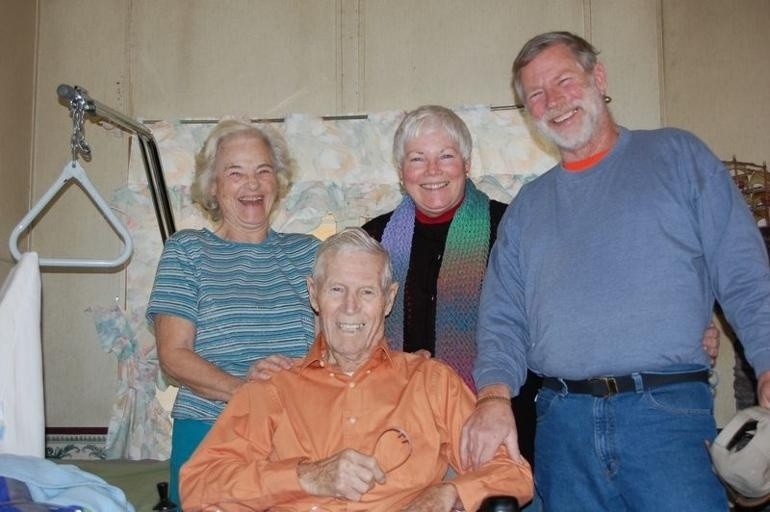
[0,251,49,464]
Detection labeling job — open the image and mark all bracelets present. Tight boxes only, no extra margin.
[474,395,513,407]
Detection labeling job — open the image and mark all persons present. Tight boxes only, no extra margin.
[455,32,770,509]
[142,116,324,511]
[179,226,536,511]
[356,104,721,511]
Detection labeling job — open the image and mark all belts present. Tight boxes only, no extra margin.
[537,369,709,398]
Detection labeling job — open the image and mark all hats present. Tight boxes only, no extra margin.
[708,405,770,509]
